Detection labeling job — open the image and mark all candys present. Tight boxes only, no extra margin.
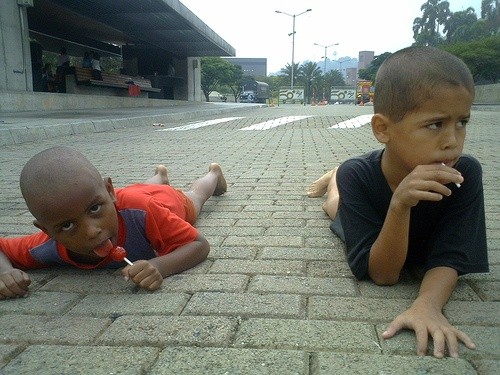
[109,246,134,266]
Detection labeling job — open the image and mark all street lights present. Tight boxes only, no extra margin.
[314,41,339,99]
[275,8,313,86]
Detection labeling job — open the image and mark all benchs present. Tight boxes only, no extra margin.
[75,67,161,93]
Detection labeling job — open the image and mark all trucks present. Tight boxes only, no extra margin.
[239,80,269,102]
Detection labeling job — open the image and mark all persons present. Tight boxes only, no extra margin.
[41,46,104,81]
[306,44,491,360]
[0,143,229,305]
